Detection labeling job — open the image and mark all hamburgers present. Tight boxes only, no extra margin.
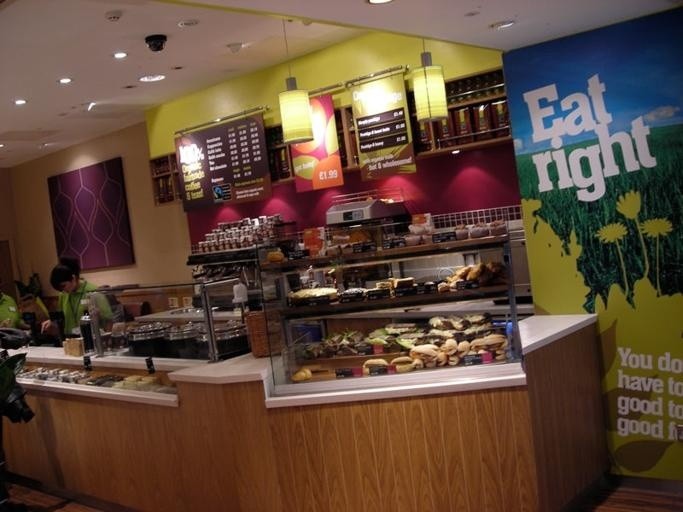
[363,315,508,375]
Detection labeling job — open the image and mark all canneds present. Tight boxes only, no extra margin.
[198,214,280,254]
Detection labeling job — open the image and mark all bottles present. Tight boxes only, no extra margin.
[64,315,95,357]
[198,214,282,253]
[158,176,174,203]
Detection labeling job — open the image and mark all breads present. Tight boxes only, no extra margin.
[284,259,505,306]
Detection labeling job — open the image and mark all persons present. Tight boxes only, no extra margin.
[39,262,114,347]
[0,289,31,329]
[179,135,204,164]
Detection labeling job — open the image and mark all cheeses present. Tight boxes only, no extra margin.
[115,373,157,395]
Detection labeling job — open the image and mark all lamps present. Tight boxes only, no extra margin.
[277,20,449,144]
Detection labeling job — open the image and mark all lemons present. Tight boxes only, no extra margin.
[293,367,311,381]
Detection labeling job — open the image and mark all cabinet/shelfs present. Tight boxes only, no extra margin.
[256,231,528,387]
[144,65,512,208]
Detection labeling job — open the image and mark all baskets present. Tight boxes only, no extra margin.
[243,308,287,357]
